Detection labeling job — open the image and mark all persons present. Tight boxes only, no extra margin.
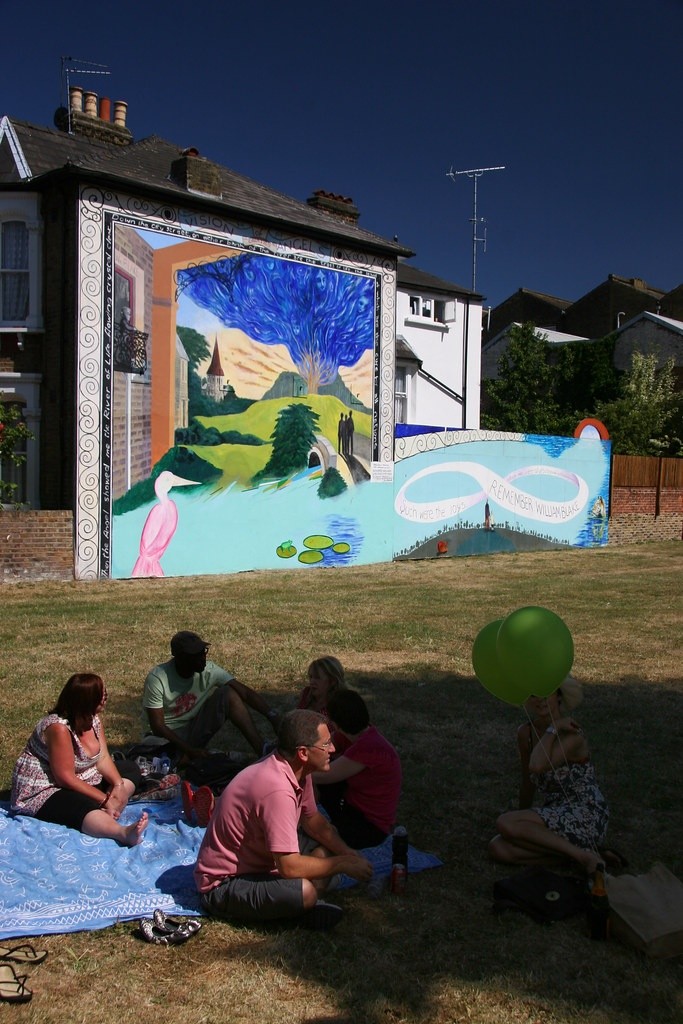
[479,671,610,889]
[193,709,374,929]
[10,673,149,848]
[140,630,402,851]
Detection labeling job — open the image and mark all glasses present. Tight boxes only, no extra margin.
[296,736,333,752]
[183,647,208,661]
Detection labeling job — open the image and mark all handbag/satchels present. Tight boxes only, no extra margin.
[492,863,610,927]
[603,860,683,958]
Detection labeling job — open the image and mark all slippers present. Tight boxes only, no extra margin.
[0,964,32,1004]
[0,944,49,965]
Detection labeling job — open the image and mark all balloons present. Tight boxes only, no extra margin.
[471,606,574,706]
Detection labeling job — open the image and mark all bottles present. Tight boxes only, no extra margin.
[150,753,171,775]
[592,871,610,940]
[392,833,408,883]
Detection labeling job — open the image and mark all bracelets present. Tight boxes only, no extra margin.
[266,708,280,719]
[546,726,556,734]
[100,792,110,807]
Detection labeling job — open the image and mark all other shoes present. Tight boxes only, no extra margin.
[192,786,215,827]
[141,917,193,946]
[589,861,606,897]
[299,898,344,932]
[181,779,195,822]
[154,909,203,934]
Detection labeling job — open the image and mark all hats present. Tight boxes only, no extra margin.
[171,631,212,657]
[524,673,584,716]
